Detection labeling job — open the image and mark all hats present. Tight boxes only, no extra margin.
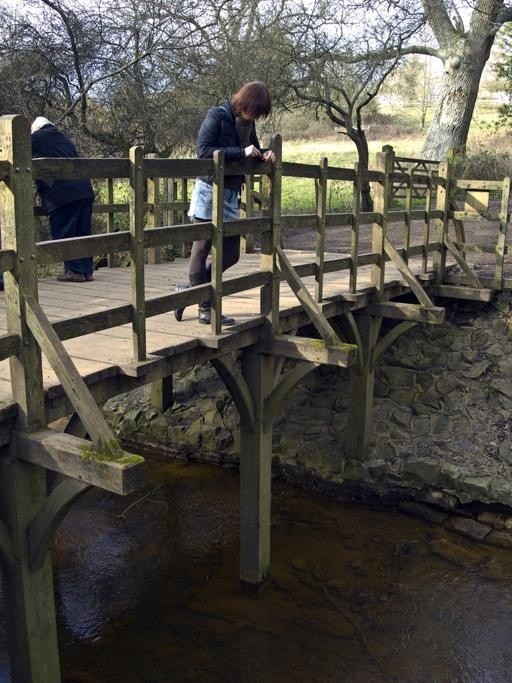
[31,117,55,133]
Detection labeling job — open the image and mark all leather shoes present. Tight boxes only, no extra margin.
[85,272,94,281]
[58,270,87,282]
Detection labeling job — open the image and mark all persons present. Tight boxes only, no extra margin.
[22,109,111,281]
[160,71,275,326]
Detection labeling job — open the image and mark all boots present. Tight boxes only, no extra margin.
[174,284,191,321]
[189,270,234,325]
